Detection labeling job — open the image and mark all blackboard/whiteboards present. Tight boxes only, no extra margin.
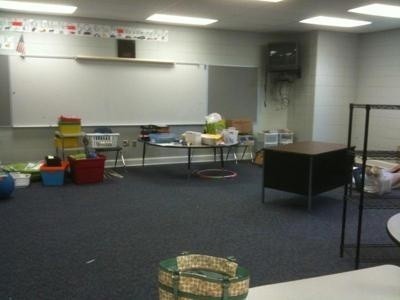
[0,54,258,127]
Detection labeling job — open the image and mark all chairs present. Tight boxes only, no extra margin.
[93,126,128,174]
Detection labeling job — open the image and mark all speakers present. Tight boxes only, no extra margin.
[117,40,135,58]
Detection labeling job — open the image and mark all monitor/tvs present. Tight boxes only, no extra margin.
[266,42,299,72]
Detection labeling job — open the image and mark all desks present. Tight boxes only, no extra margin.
[138,137,248,180]
[261,141,356,212]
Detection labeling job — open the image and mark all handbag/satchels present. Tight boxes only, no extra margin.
[158,254,249,300]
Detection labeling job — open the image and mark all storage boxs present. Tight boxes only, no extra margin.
[40,120,119,187]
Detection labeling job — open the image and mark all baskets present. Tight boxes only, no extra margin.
[85,133,119,148]
